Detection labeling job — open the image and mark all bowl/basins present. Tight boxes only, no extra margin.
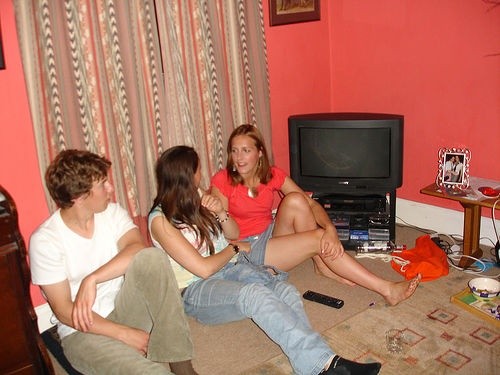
[468,277,500,298]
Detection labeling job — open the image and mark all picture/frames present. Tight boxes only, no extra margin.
[267,0,320,26]
[435,146,471,189]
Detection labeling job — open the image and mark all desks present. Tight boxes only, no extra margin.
[420,183,500,270]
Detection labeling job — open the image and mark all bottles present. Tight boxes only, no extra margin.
[355,240,407,254]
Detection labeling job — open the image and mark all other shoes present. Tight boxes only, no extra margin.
[323,355,382,375]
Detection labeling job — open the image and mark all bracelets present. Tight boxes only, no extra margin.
[215,210,229,223]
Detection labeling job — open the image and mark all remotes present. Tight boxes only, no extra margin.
[303,290,344,309]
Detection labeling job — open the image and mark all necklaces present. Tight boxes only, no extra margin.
[245,177,259,198]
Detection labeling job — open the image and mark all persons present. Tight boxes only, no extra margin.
[446,156,457,182]
[452,155,462,183]
[147,145,382,375]
[210,123,422,306]
[28,148,199,375]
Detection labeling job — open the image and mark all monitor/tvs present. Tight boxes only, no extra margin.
[287,112,403,193]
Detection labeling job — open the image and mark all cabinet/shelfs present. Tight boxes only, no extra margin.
[311,192,396,251]
[0,187,54,375]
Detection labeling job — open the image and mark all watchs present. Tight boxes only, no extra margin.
[229,243,239,254]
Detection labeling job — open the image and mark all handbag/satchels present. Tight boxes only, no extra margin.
[393,234,449,282]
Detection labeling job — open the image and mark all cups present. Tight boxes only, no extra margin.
[385,328,404,353]
